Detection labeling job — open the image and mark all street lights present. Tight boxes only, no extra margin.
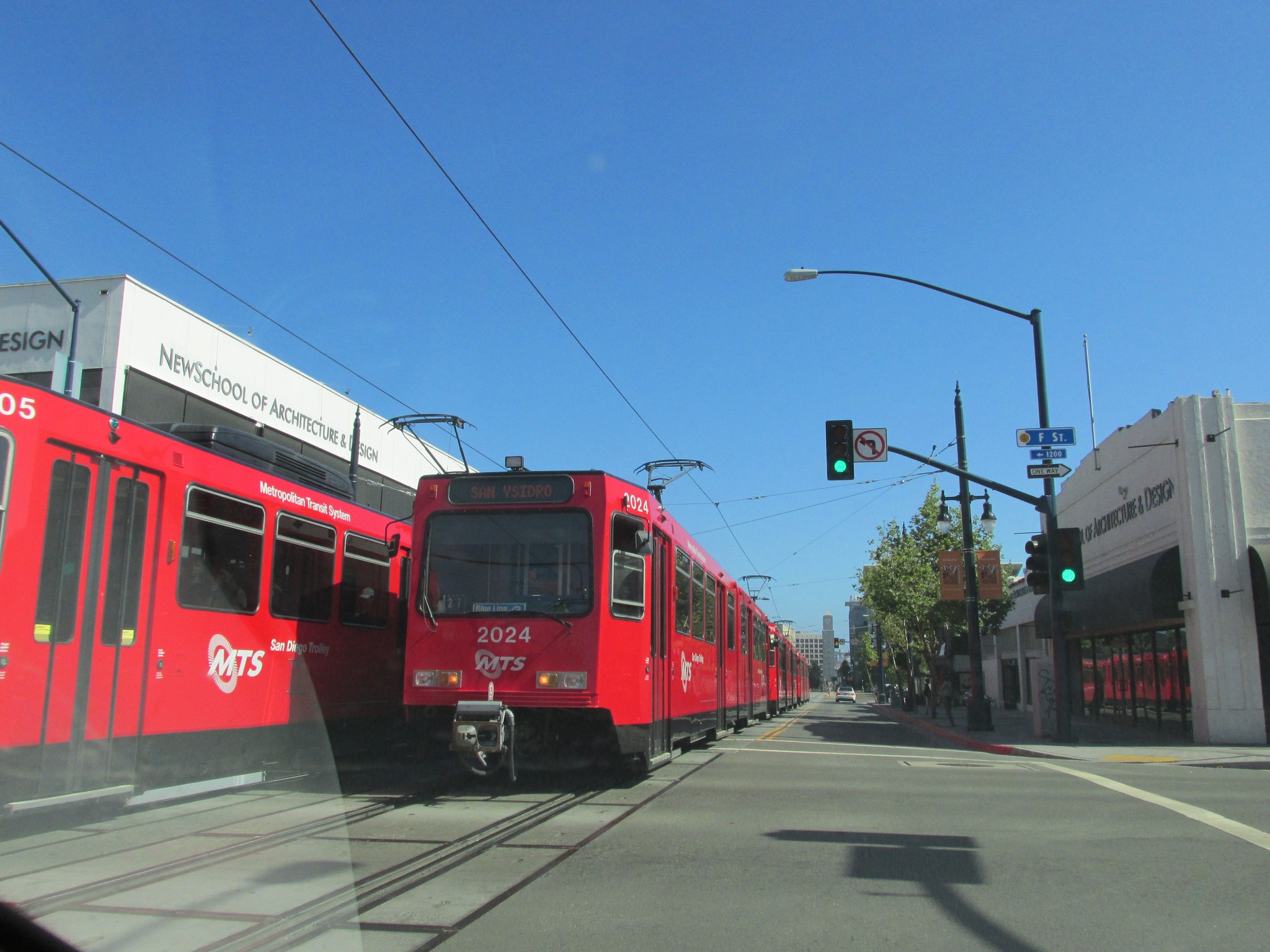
[935,380,997,731]
[870,619,890,704]
[862,615,887,704]
[783,266,1074,745]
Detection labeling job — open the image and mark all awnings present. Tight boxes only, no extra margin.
[1034,546,1185,638]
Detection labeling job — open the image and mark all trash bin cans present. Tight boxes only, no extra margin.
[891,693,901,708]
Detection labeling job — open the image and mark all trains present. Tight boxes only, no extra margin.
[1079,649,1195,716]
[0,369,469,817]
[395,459,815,788]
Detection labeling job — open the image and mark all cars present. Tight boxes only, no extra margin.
[835,687,856,703]
[874,684,906,699]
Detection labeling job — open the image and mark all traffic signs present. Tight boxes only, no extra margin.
[1027,463,1072,479]
[1029,448,1067,460]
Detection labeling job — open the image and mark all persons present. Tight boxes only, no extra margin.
[923,678,931,716]
[829,687,831,697]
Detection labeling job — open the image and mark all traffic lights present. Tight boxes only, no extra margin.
[1055,528,1085,591]
[1024,533,1050,596]
[834,638,839,648]
[825,419,854,481]
